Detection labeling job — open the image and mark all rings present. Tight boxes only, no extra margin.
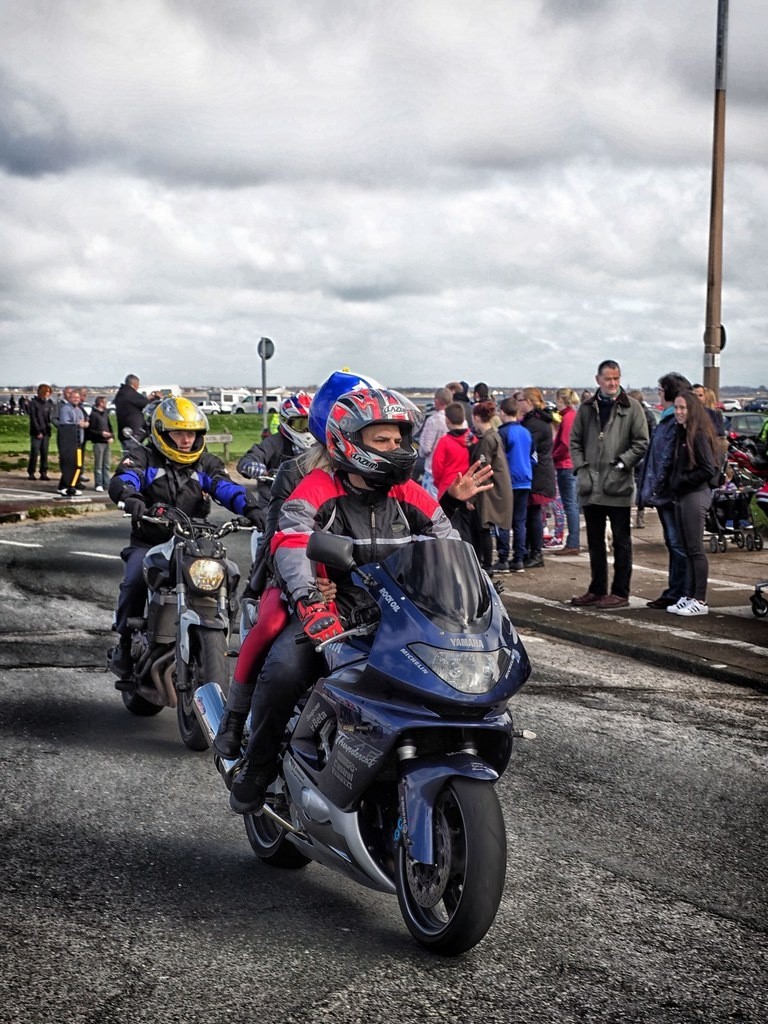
[476,479,481,486]
[473,474,477,480]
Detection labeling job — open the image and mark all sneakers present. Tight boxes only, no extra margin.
[678,599,709,616]
[229,760,269,815]
[666,595,690,613]
[212,708,246,761]
[507,560,525,573]
[491,562,510,573]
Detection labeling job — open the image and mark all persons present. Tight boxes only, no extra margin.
[569,360,722,616]
[418,382,581,574]
[48,386,115,496]
[111,374,149,457]
[9,394,16,414]
[210,368,494,816]
[19,395,31,416]
[108,396,268,678]
[26,383,52,481]
[257,398,263,413]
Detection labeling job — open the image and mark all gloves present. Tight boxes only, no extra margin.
[242,461,267,481]
[300,595,346,644]
[242,503,268,534]
[123,497,146,522]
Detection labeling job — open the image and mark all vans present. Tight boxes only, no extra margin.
[209,391,283,414]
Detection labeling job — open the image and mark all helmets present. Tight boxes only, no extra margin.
[150,396,210,464]
[307,370,389,447]
[279,392,319,450]
[325,388,423,486]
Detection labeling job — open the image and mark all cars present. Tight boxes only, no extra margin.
[644,402,663,422]
[722,399,743,412]
[744,399,768,414]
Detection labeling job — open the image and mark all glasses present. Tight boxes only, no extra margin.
[287,417,310,434]
[658,388,665,395]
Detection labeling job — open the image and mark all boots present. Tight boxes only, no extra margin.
[636,510,645,527]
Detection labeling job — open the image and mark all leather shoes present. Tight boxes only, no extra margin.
[571,591,601,607]
[524,550,543,568]
[596,594,629,607]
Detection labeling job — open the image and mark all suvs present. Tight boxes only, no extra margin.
[81,402,92,414]
[106,401,116,416]
[721,415,768,451]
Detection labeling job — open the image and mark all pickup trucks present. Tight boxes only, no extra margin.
[197,400,222,414]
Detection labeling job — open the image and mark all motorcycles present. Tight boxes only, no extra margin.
[106,465,532,958]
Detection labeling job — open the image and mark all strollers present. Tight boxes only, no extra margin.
[706,450,768,553]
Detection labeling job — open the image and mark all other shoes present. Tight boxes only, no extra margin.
[741,519,753,528]
[646,594,678,609]
[553,546,579,556]
[543,535,563,546]
[28,470,112,496]
[107,643,133,681]
[725,520,734,530]
